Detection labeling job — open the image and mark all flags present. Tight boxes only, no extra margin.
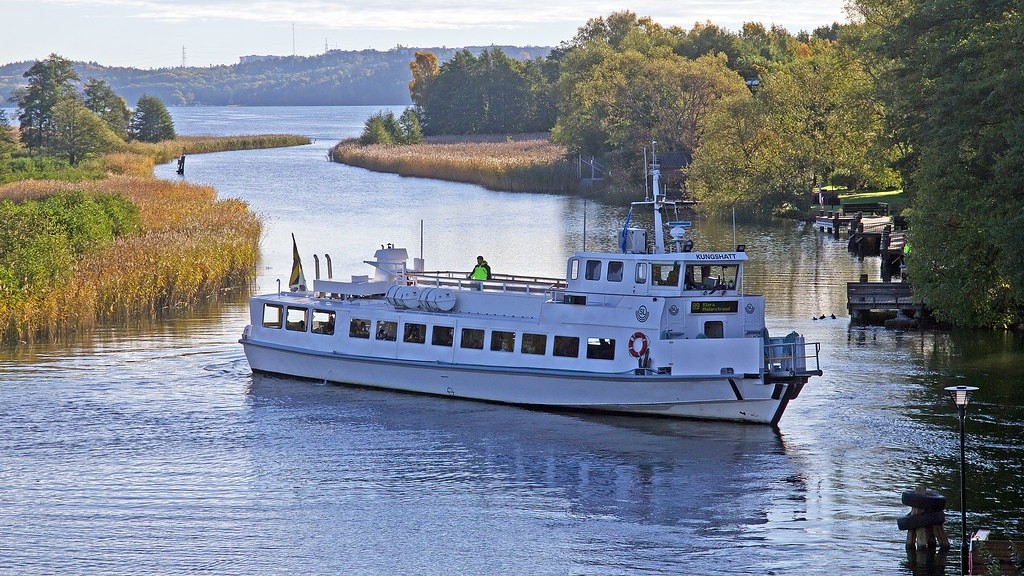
[622,206,632,254]
[288,240,303,288]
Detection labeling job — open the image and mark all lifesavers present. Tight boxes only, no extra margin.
[627,331,647,358]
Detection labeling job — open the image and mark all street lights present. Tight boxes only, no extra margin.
[944,386,980,576]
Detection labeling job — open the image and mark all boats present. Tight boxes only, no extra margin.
[237,143,822,426]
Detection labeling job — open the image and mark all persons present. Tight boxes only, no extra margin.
[669,265,697,290]
[179,154,185,169]
[899,234,912,267]
[467,256,492,281]
